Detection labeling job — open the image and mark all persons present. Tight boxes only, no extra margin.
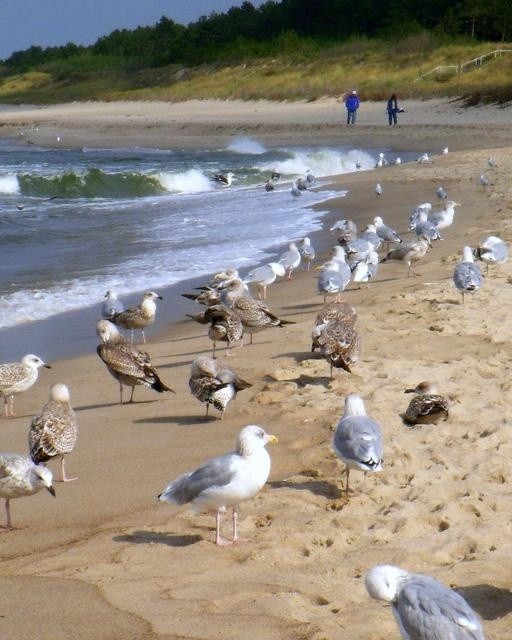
[387,94,398,125]
[345,91,359,124]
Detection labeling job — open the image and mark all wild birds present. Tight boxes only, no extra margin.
[278,243,302,280]
[415,153,428,162]
[356,160,362,169]
[486,159,500,170]
[203,167,237,189]
[177,263,296,361]
[308,318,362,383]
[311,302,359,329]
[156,422,283,549]
[185,354,255,422]
[317,199,462,301]
[376,153,388,168]
[478,231,506,268]
[451,242,484,303]
[0,455,61,534]
[290,170,317,196]
[479,174,491,185]
[434,186,449,198]
[1,351,54,424]
[333,392,385,493]
[402,381,450,426]
[271,169,281,183]
[364,563,496,640]
[96,288,175,406]
[25,382,83,486]
[264,178,274,192]
[300,236,317,270]
[372,183,385,192]
[395,157,401,165]
